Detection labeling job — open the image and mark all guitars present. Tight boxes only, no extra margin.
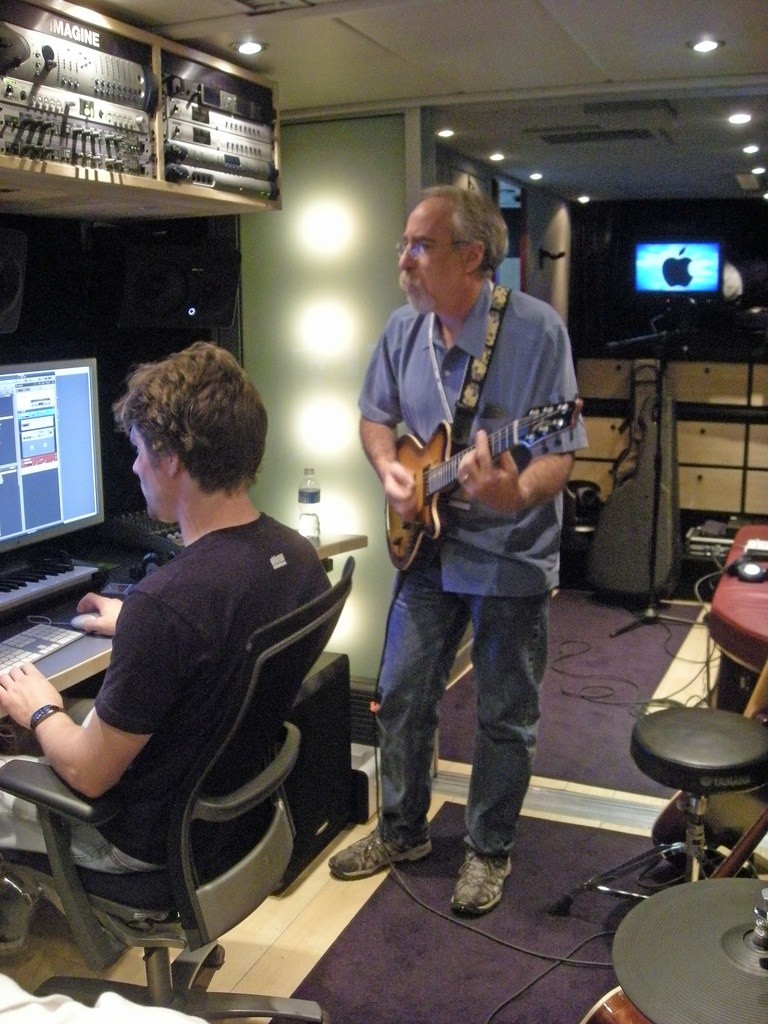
[387,391,585,574]
[579,809,768,1024]
[651,660,768,874]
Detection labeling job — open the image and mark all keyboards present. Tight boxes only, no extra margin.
[0,623,85,675]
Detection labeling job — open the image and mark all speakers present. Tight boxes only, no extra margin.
[67,238,242,330]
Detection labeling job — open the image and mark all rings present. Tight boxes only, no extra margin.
[464,474,469,480]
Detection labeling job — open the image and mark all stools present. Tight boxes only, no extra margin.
[549,703,767,918]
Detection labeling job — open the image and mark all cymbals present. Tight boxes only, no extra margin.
[610,877,768,1024]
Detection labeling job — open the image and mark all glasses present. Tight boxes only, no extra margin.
[396,241,473,260]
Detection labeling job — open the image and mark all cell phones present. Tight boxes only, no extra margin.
[100,583,137,600]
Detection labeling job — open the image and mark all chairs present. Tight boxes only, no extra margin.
[0,555,359,1024]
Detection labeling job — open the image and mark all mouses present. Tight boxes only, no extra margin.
[70,612,102,634]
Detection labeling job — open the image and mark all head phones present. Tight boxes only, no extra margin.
[130,549,180,580]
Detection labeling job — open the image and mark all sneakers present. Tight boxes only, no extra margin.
[448,843,512,915]
[328,824,432,880]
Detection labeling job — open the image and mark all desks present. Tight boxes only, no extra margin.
[709,526,768,675]
[0,534,366,716]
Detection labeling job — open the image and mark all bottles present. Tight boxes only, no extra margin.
[298,468,320,538]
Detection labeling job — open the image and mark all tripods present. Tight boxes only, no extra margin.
[606,296,709,638]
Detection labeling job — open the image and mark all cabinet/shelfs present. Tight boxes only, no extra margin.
[0,0,283,220]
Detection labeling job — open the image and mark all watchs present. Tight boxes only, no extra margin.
[31,704,64,731]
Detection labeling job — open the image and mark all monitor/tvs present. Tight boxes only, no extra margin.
[0,358,106,553]
[629,235,724,298]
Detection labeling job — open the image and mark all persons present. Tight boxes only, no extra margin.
[327,184,590,912]
[0,340,333,950]
